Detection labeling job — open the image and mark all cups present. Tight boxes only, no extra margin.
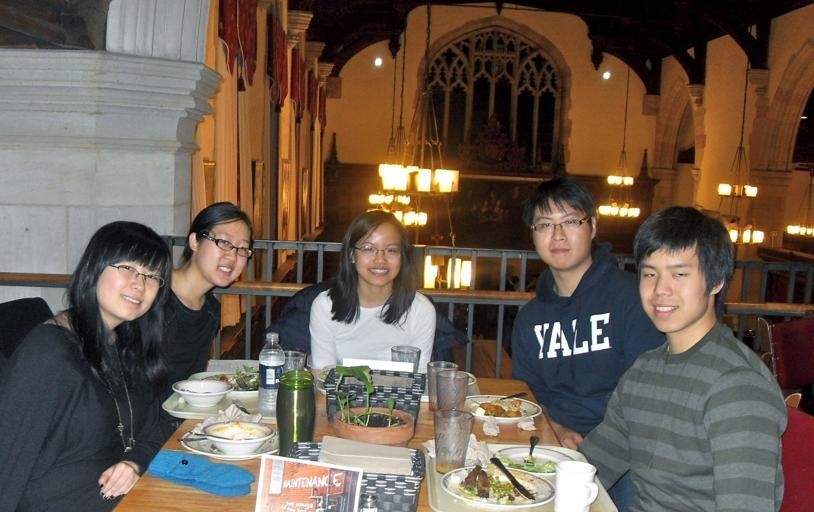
[283,350,307,374]
[427,360,458,411]
[554,461,599,512]
[391,345,421,374]
[436,370,469,416]
[433,409,475,475]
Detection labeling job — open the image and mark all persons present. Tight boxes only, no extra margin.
[510,176,661,511]
[147,202,254,430]
[0,220,171,511]
[574,206,788,512]
[309,211,437,374]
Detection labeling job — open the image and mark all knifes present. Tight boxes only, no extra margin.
[489,457,535,500]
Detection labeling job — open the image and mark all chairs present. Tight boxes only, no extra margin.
[757,309,814,388]
[777,392,814,512]
[0,296,54,365]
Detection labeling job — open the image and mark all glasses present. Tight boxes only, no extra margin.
[202,233,253,258]
[109,265,166,289]
[529,215,592,232]
[354,246,399,253]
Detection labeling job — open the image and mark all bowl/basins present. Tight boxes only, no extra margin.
[172,379,234,408]
[203,421,276,454]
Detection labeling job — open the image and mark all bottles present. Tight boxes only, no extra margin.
[257,333,286,419]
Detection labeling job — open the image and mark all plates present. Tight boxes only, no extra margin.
[464,394,542,423]
[205,373,262,398]
[439,465,555,509]
[459,370,476,386]
[181,426,280,462]
[494,446,575,476]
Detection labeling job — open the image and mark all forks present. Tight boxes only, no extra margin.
[499,392,528,402]
[524,436,540,465]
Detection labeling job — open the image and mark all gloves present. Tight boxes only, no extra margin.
[145,449,256,497]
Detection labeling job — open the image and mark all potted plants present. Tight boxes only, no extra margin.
[331,365,415,449]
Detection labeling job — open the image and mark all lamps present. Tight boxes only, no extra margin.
[596,64,641,217]
[717,27,764,245]
[786,172,814,238]
[364,36,428,214]
[377,2,475,290]
[377,16,430,227]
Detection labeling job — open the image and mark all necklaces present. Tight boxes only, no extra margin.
[70,327,136,456]
